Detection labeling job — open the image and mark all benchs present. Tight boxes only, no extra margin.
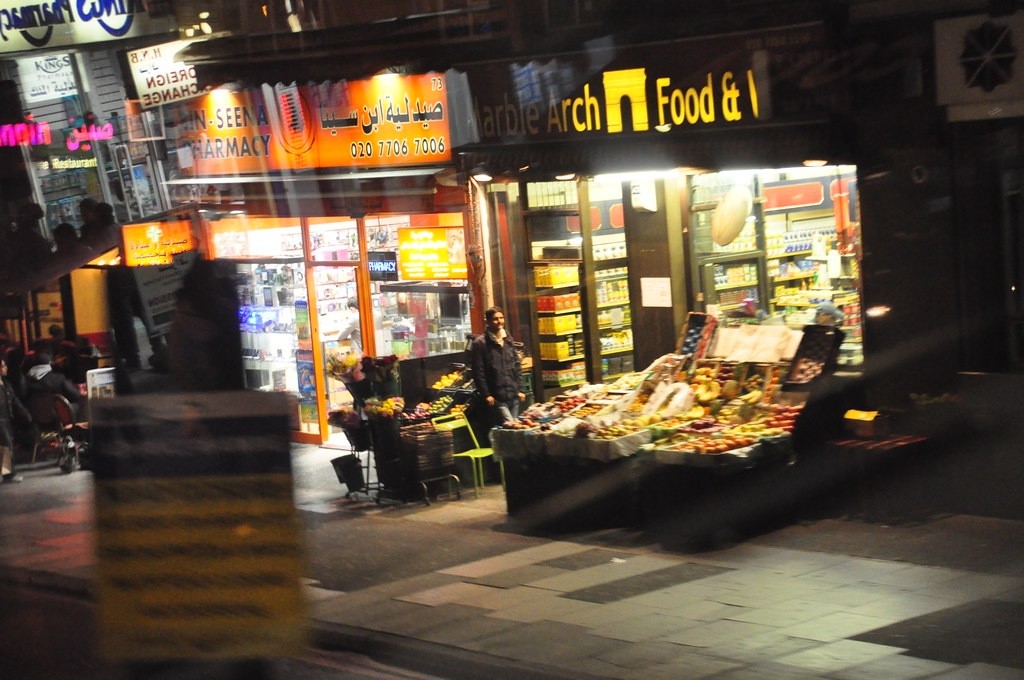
[825,430,928,511]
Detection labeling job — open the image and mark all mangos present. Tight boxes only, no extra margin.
[746,370,780,403]
[718,366,737,388]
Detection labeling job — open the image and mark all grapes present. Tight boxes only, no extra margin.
[575,421,597,438]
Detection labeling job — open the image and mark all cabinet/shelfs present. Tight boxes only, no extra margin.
[714,242,819,326]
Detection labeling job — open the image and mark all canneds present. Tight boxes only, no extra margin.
[766,226,836,256]
[713,264,729,288]
[842,302,862,326]
[595,279,629,304]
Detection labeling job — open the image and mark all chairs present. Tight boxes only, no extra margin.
[431,412,507,501]
[2,375,91,472]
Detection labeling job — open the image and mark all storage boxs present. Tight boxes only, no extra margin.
[532,262,588,392]
[780,323,846,393]
[675,310,715,361]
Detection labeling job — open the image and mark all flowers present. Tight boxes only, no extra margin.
[321,355,406,430]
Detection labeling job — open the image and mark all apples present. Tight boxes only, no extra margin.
[598,402,803,453]
[503,397,599,437]
[402,412,430,420]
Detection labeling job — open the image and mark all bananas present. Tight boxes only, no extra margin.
[692,367,720,402]
[740,390,762,404]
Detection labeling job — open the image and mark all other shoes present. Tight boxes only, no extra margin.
[12,475,23,482]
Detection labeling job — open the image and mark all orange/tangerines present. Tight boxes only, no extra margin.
[376,370,468,415]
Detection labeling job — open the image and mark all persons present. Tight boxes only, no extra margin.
[473,306,526,423]
[0,359,36,481]
[339,298,363,355]
[27,323,95,423]
[0,194,123,288]
[816,303,844,325]
